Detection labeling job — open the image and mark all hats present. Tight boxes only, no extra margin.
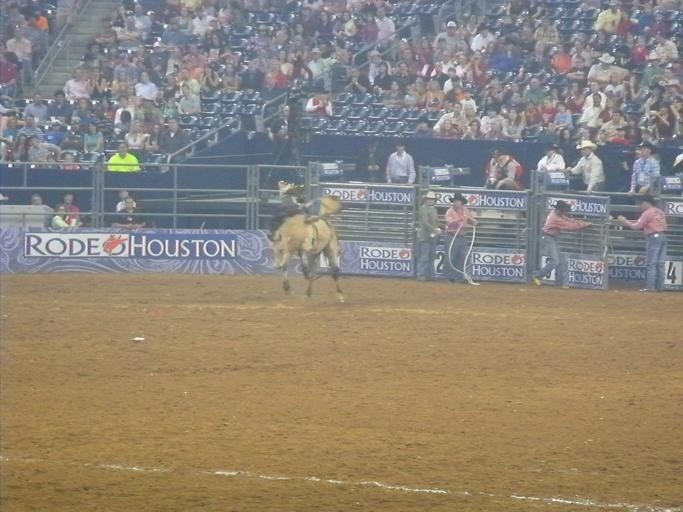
[544,143,561,150]
[576,139,599,153]
[551,200,573,212]
[636,141,655,155]
[634,193,658,205]
[423,191,467,204]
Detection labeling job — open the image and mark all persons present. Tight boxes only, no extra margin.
[411,191,444,282]
[530,199,592,290]
[443,192,478,285]
[0,2,682,241]
[616,195,668,293]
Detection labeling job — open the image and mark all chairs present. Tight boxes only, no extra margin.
[0,1,683,172]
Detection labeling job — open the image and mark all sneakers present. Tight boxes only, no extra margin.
[533,275,541,286]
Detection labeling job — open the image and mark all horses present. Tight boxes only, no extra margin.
[268,195,346,304]
